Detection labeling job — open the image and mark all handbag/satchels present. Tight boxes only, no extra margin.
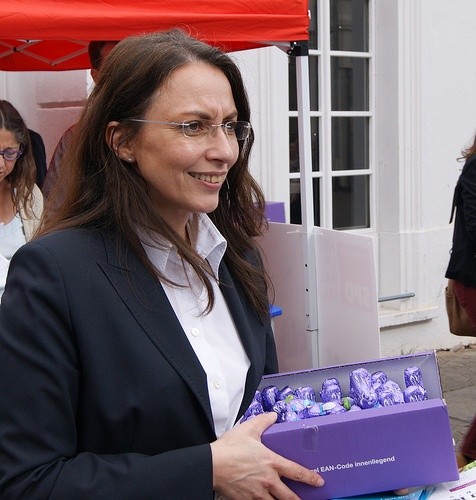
[445,278,476,337]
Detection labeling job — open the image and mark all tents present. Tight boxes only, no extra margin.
[0,0,320,362]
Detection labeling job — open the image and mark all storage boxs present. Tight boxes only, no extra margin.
[237,349,459,500]
[254,200,285,224]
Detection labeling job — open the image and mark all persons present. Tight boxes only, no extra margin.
[0,32,413,500]
[0,100,43,296]
[28,129,46,186]
[41,39,125,199]
[446,132,476,469]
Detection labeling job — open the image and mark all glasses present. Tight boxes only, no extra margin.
[0,142,23,161]
[122,115,251,141]
